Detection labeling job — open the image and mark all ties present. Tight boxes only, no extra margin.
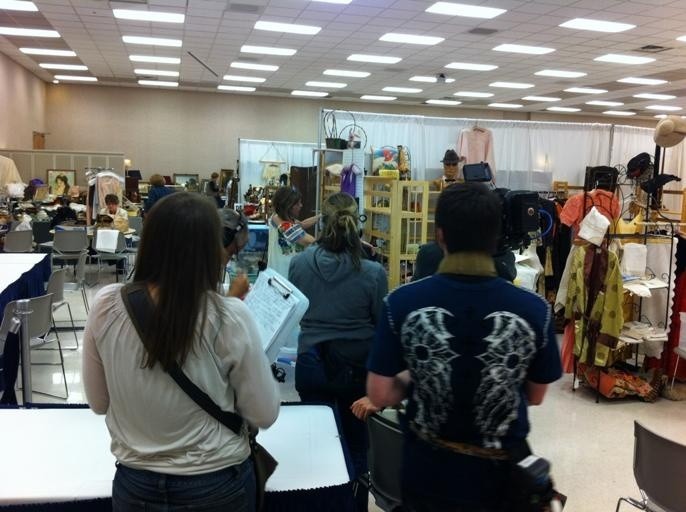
[445,178,456,182]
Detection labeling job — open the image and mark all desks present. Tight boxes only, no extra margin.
[0,402,352,509]
[0,252,51,340]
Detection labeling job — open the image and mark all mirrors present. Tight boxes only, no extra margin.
[320,149,343,205]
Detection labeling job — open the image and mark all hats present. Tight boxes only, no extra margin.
[626,152,650,179]
[653,115,686,147]
[440,149,463,163]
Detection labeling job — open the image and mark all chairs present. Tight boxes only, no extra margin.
[128,215,142,273]
[92,230,127,284]
[33,266,79,351]
[50,229,91,286]
[46,248,89,314]
[4,229,34,253]
[368,410,567,512]
[616,420,686,512]
[1,293,68,399]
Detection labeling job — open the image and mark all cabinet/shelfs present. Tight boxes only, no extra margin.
[363,177,429,291]
[573,164,674,404]
[427,179,443,240]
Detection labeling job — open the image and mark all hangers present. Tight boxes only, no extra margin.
[588,180,599,194]
[259,142,287,164]
[653,222,680,232]
[473,120,485,133]
[571,236,610,253]
[539,189,565,199]
[87,168,123,186]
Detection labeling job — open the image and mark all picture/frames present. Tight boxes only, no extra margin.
[47,169,76,197]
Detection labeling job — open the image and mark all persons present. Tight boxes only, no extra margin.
[266,185,325,279]
[81,191,280,511]
[366,181,561,510]
[287,191,388,509]
[22,172,252,299]
[434,149,466,191]
[350,395,380,422]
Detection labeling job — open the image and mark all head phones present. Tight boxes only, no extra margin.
[222,210,247,248]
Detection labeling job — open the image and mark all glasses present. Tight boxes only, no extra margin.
[230,235,239,262]
[279,185,297,204]
[445,163,458,166]
